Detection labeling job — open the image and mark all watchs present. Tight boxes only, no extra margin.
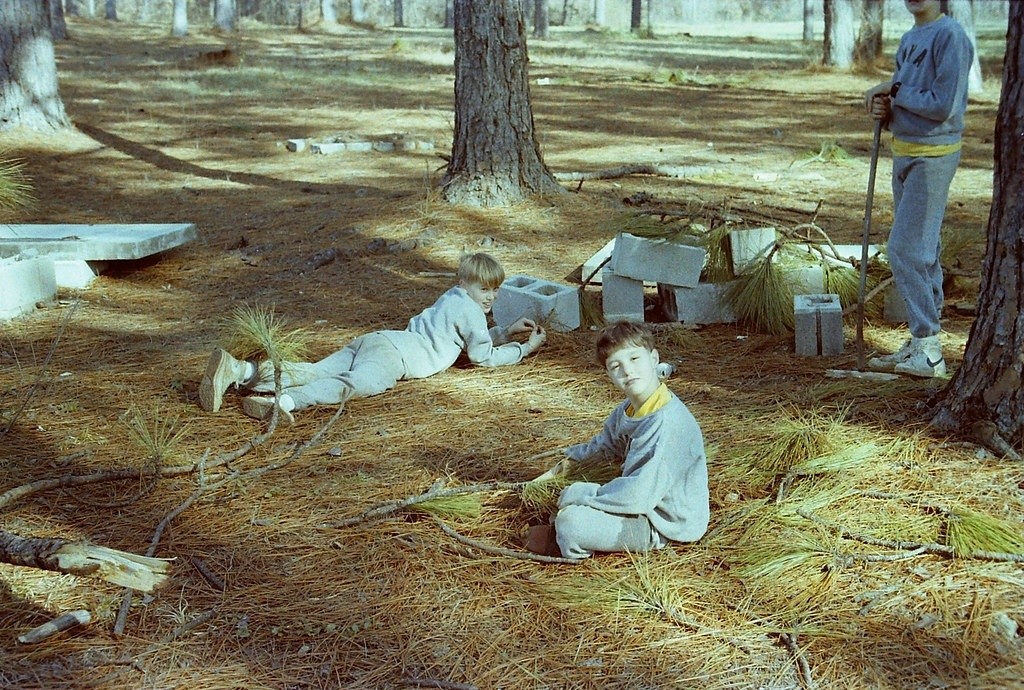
[891,81,902,98]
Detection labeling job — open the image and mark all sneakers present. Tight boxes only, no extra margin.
[199,349,247,413]
[243,397,294,428]
[868,334,946,378]
[521,524,561,557]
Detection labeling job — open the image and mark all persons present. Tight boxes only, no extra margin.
[201,253,548,426]
[862,0,975,378]
[519,320,710,558]
